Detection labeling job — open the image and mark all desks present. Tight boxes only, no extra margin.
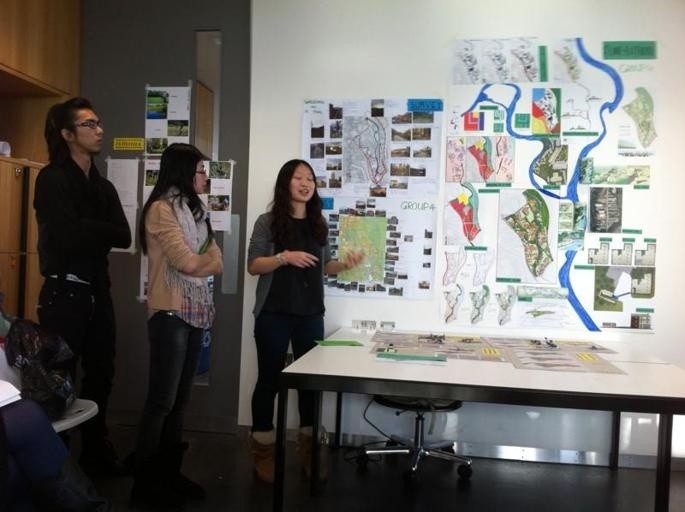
[275,326,685,512]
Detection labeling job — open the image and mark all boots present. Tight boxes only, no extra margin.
[299,431,329,485]
[248,431,290,486]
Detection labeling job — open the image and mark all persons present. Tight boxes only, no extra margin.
[0,311,78,479]
[134,141,225,471]
[276,251,287,267]
[32,95,132,428]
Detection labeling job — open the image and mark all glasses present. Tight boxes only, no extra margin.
[196,169,207,175]
[73,120,103,129]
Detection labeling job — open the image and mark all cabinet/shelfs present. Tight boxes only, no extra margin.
[0,1,79,96]
[21,167,45,326]
[0,160,22,318]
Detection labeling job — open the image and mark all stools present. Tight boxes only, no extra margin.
[357,393,472,489]
[51,398,101,439]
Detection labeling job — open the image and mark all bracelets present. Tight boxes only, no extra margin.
[246,158,365,484]
